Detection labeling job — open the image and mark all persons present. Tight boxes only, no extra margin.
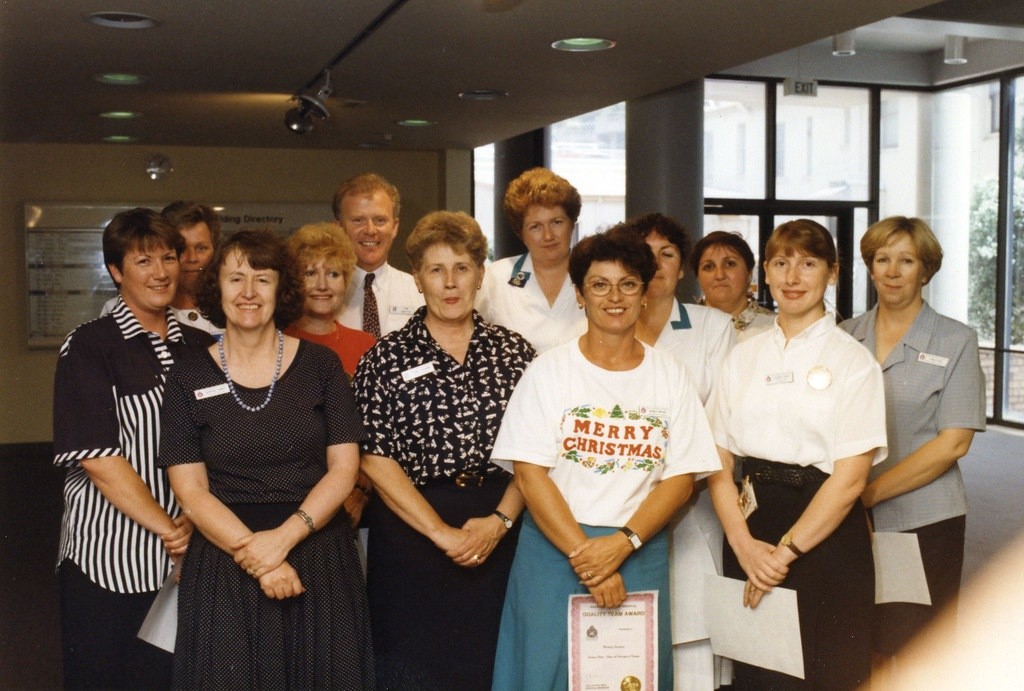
[284,222,379,586]
[473,168,589,354]
[351,209,539,691]
[159,200,221,309]
[330,172,427,337]
[159,222,377,691]
[703,216,889,691]
[628,212,733,691]
[53,207,217,691]
[489,223,726,691]
[837,216,987,675]
[690,230,776,486]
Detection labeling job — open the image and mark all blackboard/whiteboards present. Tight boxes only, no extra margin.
[22,204,333,349]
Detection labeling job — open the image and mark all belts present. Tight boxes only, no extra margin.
[741,457,829,489]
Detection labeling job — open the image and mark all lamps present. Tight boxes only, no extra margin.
[832,29,856,57]
[284,69,333,135]
[943,34,968,65]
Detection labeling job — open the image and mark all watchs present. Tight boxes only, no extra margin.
[781,532,804,556]
[619,526,642,551]
[495,510,513,528]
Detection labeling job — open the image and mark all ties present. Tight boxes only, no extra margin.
[363,273,381,342]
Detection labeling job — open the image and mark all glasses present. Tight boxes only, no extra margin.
[582,275,645,296]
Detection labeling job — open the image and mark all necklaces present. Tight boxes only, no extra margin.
[218,327,284,412]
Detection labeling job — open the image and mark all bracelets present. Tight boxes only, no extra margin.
[355,483,372,499]
[294,509,315,536]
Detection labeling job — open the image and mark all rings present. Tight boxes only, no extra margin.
[474,555,480,562]
[586,571,592,579]
